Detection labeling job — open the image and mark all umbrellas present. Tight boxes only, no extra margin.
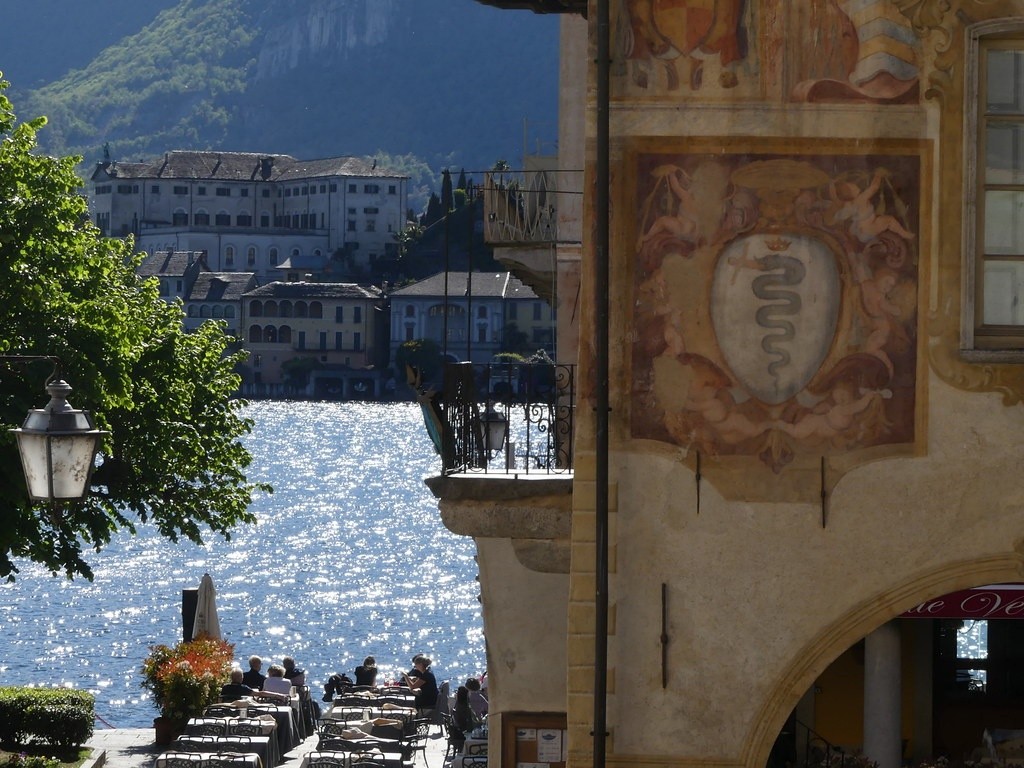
[190,573,221,641]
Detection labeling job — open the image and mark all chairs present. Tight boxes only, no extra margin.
[306,750,345,768]
[171,734,252,752]
[227,718,263,736]
[204,706,241,720]
[441,706,465,768]
[462,743,488,768]
[246,705,278,721]
[401,718,431,768]
[412,682,450,735]
[379,708,412,725]
[192,717,226,736]
[315,718,347,757]
[340,685,409,708]
[316,740,382,754]
[156,752,246,768]
[341,707,372,721]
[348,751,386,767]
[253,686,308,708]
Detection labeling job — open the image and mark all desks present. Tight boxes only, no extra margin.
[155,751,262,768]
[332,706,418,723]
[376,684,411,696]
[242,692,299,724]
[301,753,402,768]
[187,718,280,768]
[452,757,488,768]
[204,703,300,759]
[177,735,270,768]
[463,735,488,757]
[334,696,415,708]
[333,718,403,731]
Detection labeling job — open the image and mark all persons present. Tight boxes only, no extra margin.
[221,668,263,702]
[399,654,439,708]
[243,655,267,691]
[263,663,292,694]
[464,678,488,715]
[354,656,378,685]
[283,659,306,685]
[452,686,474,739]
